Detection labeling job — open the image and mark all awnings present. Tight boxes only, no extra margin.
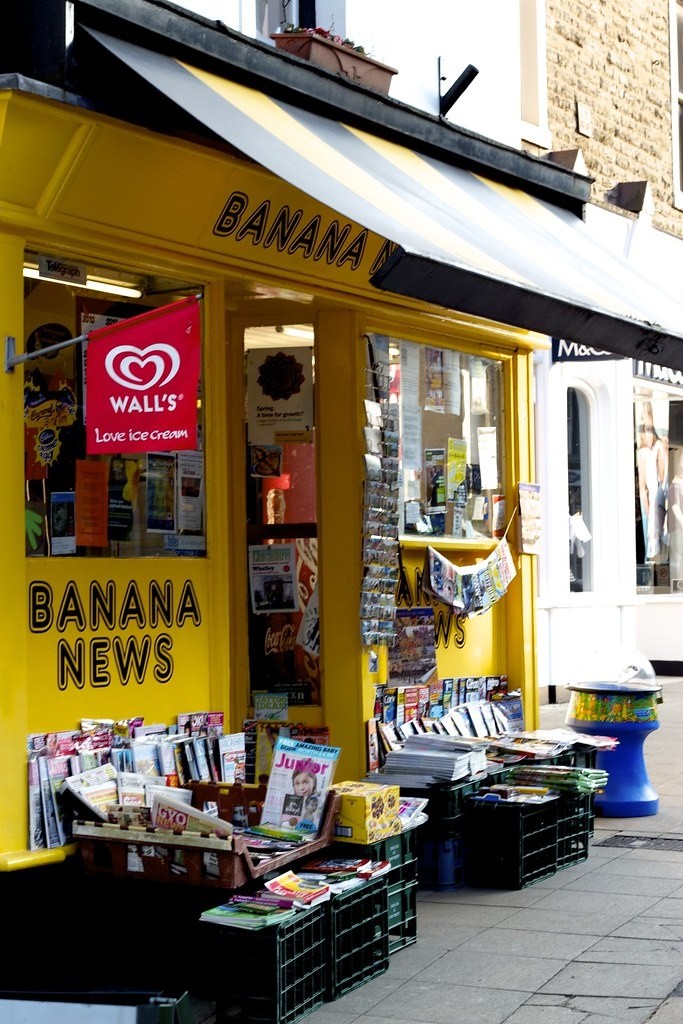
[79,21,683,370]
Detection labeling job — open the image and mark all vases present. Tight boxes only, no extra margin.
[270,33,398,95]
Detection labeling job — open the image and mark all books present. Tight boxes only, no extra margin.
[368,675,568,789]
[30,711,429,931]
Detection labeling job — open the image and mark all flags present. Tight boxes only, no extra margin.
[86,296,201,454]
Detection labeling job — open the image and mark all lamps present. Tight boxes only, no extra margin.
[23,252,147,300]
[275,326,314,339]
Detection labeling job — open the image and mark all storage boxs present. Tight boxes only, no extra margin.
[401,744,599,893]
[215,832,419,1024]
[333,780,400,845]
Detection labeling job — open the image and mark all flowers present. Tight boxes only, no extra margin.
[284,14,368,56]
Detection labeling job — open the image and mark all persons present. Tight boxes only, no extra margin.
[636,401,666,517]
[303,796,318,821]
[292,770,317,796]
